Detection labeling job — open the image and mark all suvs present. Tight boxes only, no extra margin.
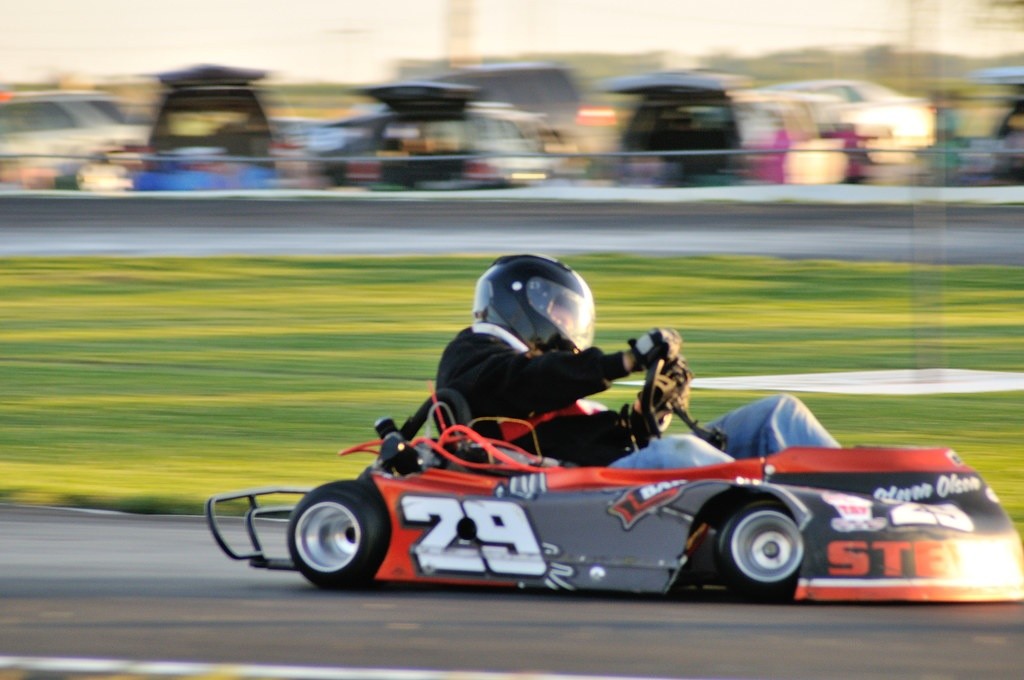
[597,67,869,188]
[352,81,579,192]
[142,63,279,180]
[960,70,1024,187]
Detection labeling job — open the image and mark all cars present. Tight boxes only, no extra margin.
[275,63,587,184]
[1,88,157,188]
[759,80,938,164]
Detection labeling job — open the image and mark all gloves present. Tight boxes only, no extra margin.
[629,327,682,372]
[638,361,692,406]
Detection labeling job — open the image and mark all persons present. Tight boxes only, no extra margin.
[770,102,818,184]
[434,252,842,468]
[132,143,282,193]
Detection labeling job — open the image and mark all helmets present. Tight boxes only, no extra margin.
[474,253,596,355]
